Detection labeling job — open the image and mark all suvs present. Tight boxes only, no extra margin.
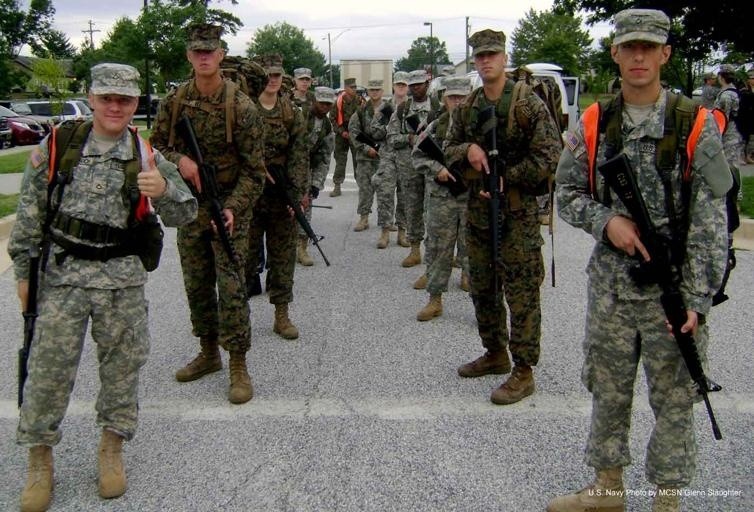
[0,100,55,136]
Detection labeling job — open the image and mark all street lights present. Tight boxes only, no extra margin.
[423,22,433,84]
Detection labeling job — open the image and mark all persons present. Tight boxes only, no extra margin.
[442,28,563,407]
[412,76,472,294]
[347,74,399,233]
[384,69,446,267]
[695,63,753,173]
[242,50,309,339]
[368,70,420,250]
[288,66,313,102]
[291,85,335,267]
[328,76,368,199]
[545,8,740,512]
[5,63,199,511]
[409,76,472,323]
[149,21,267,405]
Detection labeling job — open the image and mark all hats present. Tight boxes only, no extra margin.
[611,8,671,46]
[367,69,429,90]
[90,62,142,97]
[703,64,754,80]
[314,86,334,104]
[344,77,356,87]
[187,23,224,51]
[253,54,283,74]
[294,67,312,79]
[467,28,506,56]
[444,76,471,97]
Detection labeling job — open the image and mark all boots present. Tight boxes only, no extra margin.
[547,467,624,512]
[747,155,754,164]
[458,351,511,378]
[651,485,680,512]
[176,338,222,382]
[274,303,298,340]
[354,214,411,248]
[402,243,470,321]
[491,366,535,405]
[21,446,54,512]
[98,431,126,499]
[298,238,313,266]
[229,352,253,404]
[330,183,340,197]
[738,154,747,165]
[245,274,262,298]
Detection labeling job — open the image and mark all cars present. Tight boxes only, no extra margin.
[0,118,15,150]
[0,106,45,145]
[166,81,176,90]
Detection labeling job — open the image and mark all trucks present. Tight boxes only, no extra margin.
[133,94,162,116]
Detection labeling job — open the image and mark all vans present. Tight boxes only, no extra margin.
[23,101,95,126]
[466,63,580,140]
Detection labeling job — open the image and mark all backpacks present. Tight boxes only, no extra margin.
[718,84,754,157]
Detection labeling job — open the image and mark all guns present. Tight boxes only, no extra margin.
[19,257,38,407]
[267,165,329,266]
[355,133,381,152]
[598,152,722,441]
[417,135,467,197]
[176,116,246,298]
[476,107,503,305]
